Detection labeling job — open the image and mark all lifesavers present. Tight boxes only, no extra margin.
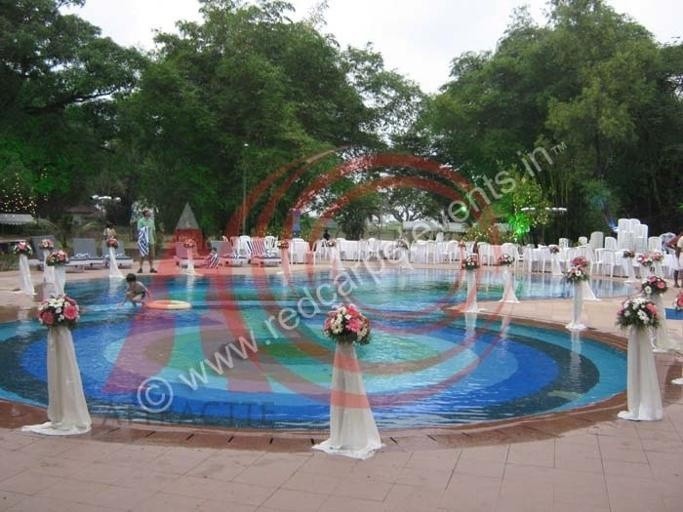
[146,299,192,310]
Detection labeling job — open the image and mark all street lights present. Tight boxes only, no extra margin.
[242,143,249,236]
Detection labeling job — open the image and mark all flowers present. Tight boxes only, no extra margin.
[13,237,120,328]
[183,238,196,248]
[324,239,337,248]
[395,236,409,249]
[323,303,374,347]
[277,239,290,249]
[461,244,682,330]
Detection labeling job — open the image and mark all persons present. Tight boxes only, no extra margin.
[665,229,682,289]
[137,209,157,273]
[117,273,152,308]
[102,222,117,240]
[322,229,329,240]
[675,232,682,287]
